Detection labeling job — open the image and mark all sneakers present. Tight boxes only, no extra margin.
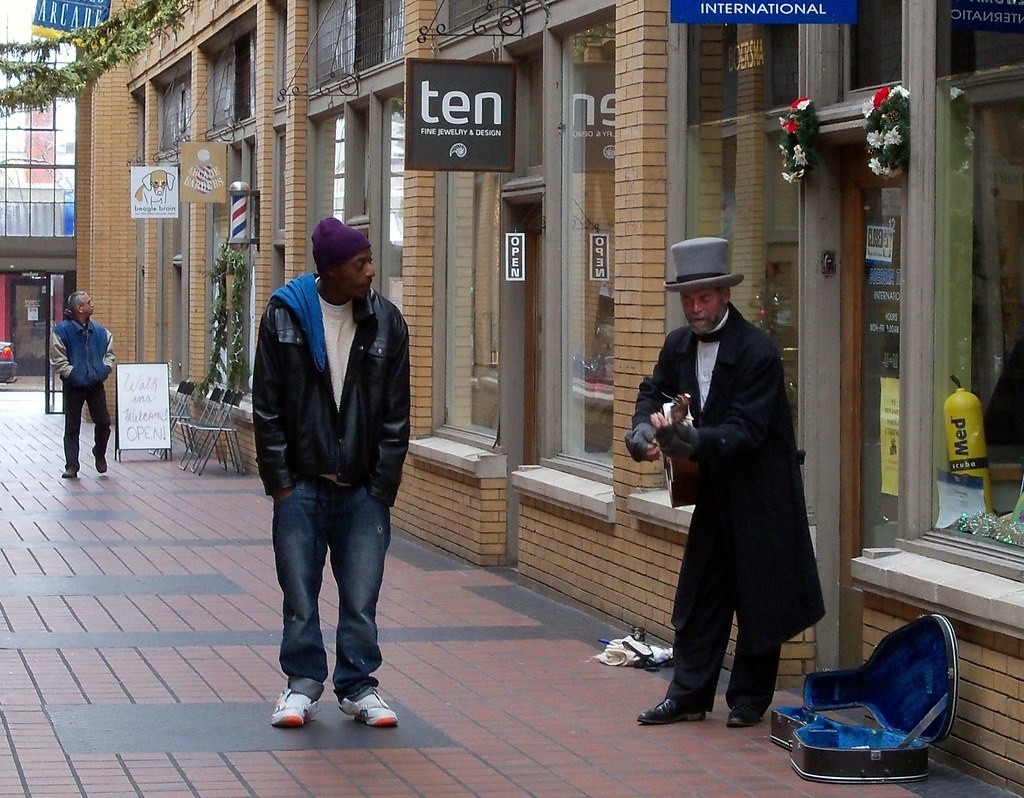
[337,685,398,726]
[271,689,319,726]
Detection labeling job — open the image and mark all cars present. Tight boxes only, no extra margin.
[0,341,18,384]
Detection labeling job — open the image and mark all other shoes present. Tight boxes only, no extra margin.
[62,465,77,478]
[92,447,107,473]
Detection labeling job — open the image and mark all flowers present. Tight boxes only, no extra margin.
[779,95,818,184]
[861,84,910,181]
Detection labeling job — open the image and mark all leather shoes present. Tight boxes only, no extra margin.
[726,704,760,727]
[636,698,706,723]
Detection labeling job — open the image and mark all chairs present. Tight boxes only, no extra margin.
[149,380,245,476]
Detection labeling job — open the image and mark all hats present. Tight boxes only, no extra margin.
[311,217,371,274]
[663,237,744,292]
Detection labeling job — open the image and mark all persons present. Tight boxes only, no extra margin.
[251,216,411,729]
[48,291,116,479]
[624,235,828,727]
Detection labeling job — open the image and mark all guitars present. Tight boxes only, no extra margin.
[661,391,702,511]
